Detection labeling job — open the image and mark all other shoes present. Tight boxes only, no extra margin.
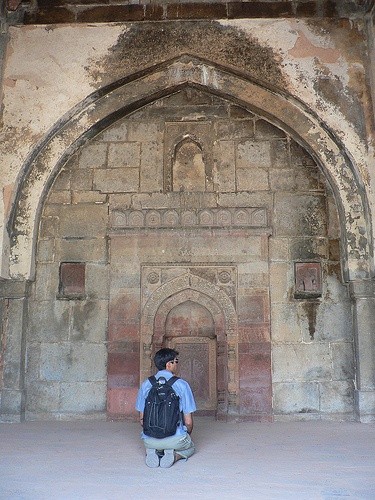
[160,449,175,467]
[146,447,159,467]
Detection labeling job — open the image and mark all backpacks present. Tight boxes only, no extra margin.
[143,375,179,438]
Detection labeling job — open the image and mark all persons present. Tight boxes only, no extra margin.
[135,347,196,469]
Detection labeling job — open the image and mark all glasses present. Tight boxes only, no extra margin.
[168,359,178,363]
